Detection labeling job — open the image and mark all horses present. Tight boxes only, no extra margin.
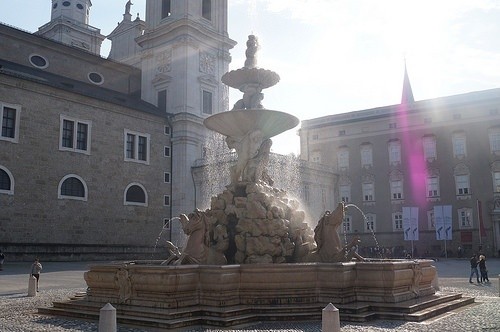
[160,209,227,266]
[307,201,362,264]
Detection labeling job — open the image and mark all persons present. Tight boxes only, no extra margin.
[0,252,6,271]
[469,254,480,284]
[32,258,42,292]
[458,246,464,259]
[478,254,490,283]
[358,246,418,258]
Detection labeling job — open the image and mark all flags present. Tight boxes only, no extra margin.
[477,201,486,236]
